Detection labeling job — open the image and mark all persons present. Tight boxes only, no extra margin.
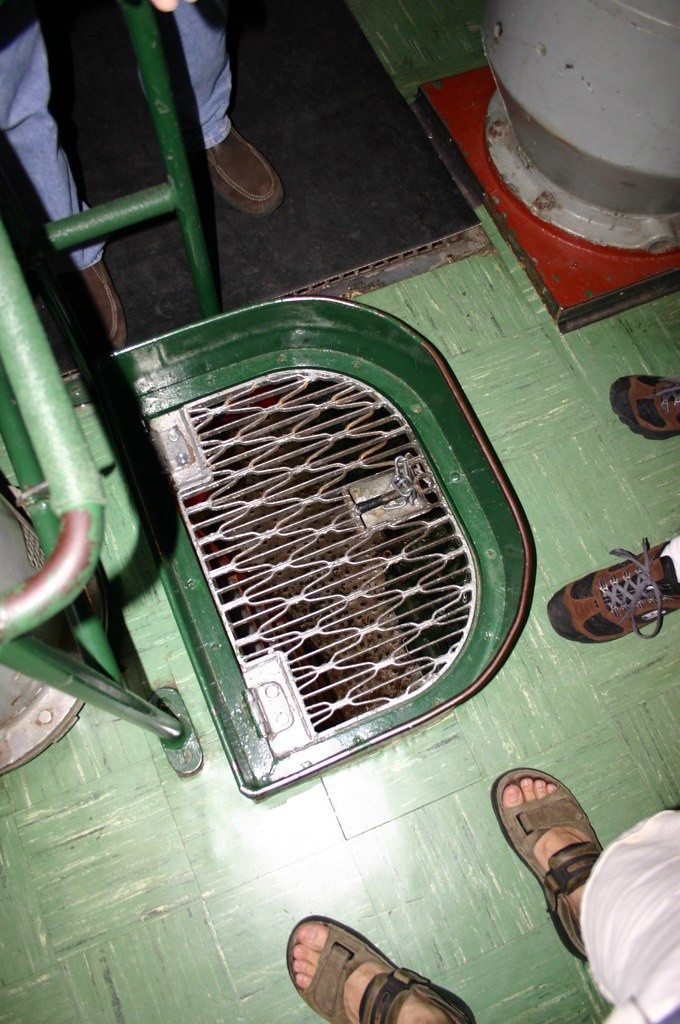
[287,770,680,1024]
[0,0,283,351]
[548,377,680,643]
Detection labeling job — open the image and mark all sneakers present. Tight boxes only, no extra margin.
[609,374,680,440]
[547,538,680,644]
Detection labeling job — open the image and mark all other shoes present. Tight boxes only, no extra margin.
[82,261,128,351]
[206,126,284,218]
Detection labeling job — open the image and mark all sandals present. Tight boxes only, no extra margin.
[492,767,605,962]
[287,915,476,1024]
